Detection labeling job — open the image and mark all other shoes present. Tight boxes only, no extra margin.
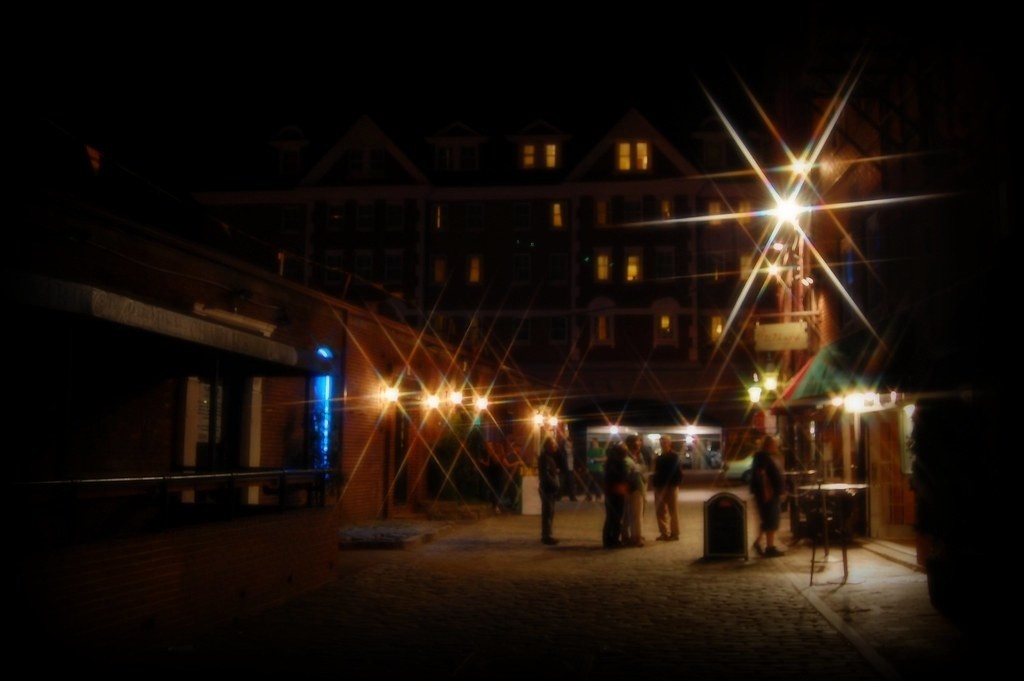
[656,533,667,541]
[766,546,783,557]
[604,540,622,548]
[669,534,679,541]
[620,537,644,547]
[540,536,557,545]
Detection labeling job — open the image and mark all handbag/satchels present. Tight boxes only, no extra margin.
[604,481,627,496]
[647,473,668,491]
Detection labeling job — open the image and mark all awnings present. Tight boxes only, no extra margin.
[771,310,949,410]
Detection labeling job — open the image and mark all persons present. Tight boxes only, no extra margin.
[480,441,522,513]
[537,438,559,544]
[653,435,681,540]
[750,436,785,557]
[557,437,578,501]
[586,438,606,500]
[603,436,650,548]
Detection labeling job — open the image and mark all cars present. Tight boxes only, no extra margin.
[722,456,754,484]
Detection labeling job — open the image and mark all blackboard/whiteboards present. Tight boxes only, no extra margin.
[704,492,748,561]
